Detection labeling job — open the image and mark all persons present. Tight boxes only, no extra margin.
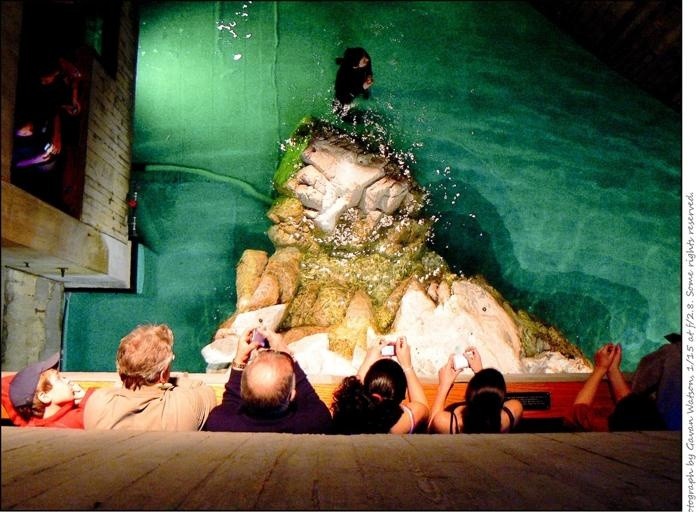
[430,345,525,434]
[571,341,667,432]
[329,336,432,434]
[334,45,375,104]
[0,351,102,429]
[31,52,85,117]
[84,325,218,433]
[202,318,335,435]
[10,105,64,182]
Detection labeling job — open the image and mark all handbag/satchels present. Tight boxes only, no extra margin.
[401,365,414,373]
[231,358,246,370]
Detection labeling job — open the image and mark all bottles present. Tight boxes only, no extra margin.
[9,351,62,408]
